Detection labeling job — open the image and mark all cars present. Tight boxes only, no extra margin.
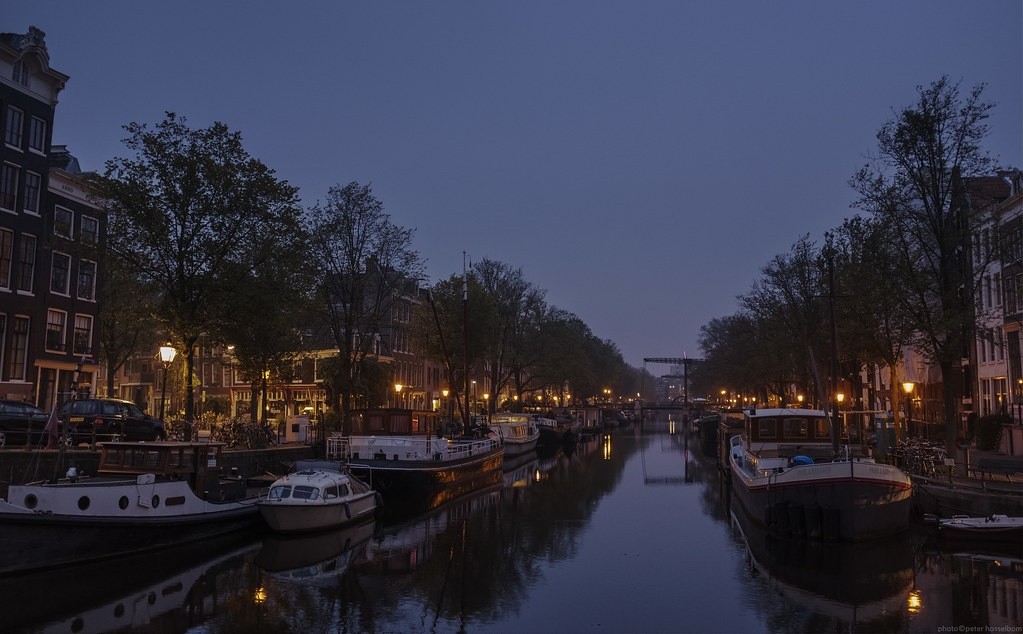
[0,399,78,450]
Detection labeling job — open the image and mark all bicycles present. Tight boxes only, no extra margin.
[210,418,278,449]
[887,436,950,483]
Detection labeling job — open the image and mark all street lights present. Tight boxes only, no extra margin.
[442,386,449,412]
[158,338,177,422]
[744,397,747,402]
[797,394,804,402]
[1019,379,1023,398]
[737,393,741,399]
[394,382,402,408]
[483,392,489,410]
[752,396,757,402]
[553,396,557,401]
[537,395,542,401]
[837,392,844,412]
[603,388,611,403]
[902,379,915,438]
[909,576,923,616]
[721,389,726,396]
[565,394,570,401]
[514,395,518,402]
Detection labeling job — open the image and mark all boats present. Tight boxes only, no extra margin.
[323,249,505,472]
[933,514,1023,560]
[0,531,268,634]
[716,409,746,470]
[255,455,382,531]
[694,414,717,428]
[270,517,376,583]
[727,493,914,622]
[729,346,912,540]
[581,407,634,429]
[536,410,583,440]
[486,411,541,454]
[0,352,266,573]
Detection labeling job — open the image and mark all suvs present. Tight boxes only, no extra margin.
[58,398,166,442]
